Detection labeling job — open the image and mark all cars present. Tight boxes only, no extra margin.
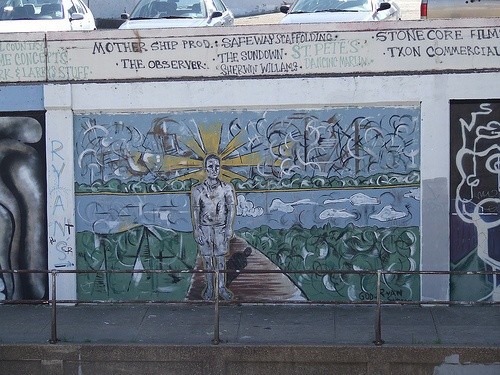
[278,0,400,24]
[0,0,98,31]
[117,0,235,30]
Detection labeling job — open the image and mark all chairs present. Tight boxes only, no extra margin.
[5,2,63,20]
[142,1,201,19]
